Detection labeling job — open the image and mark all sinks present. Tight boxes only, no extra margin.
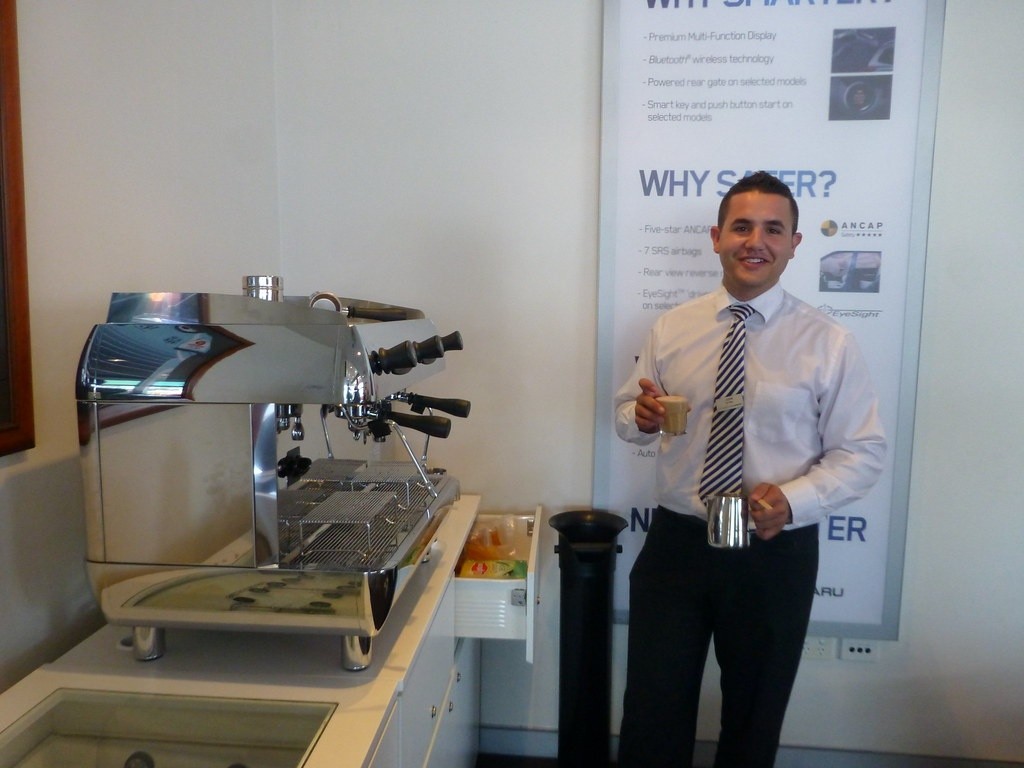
[1,685,340,767]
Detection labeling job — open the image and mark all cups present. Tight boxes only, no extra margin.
[705,494,758,548]
[657,396,688,439]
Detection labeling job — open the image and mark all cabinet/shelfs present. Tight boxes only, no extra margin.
[361,680,401,768]
[398,495,544,768]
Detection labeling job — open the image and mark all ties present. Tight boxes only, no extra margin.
[697,304,756,513]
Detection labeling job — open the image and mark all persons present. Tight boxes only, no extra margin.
[614,171,887,768]
